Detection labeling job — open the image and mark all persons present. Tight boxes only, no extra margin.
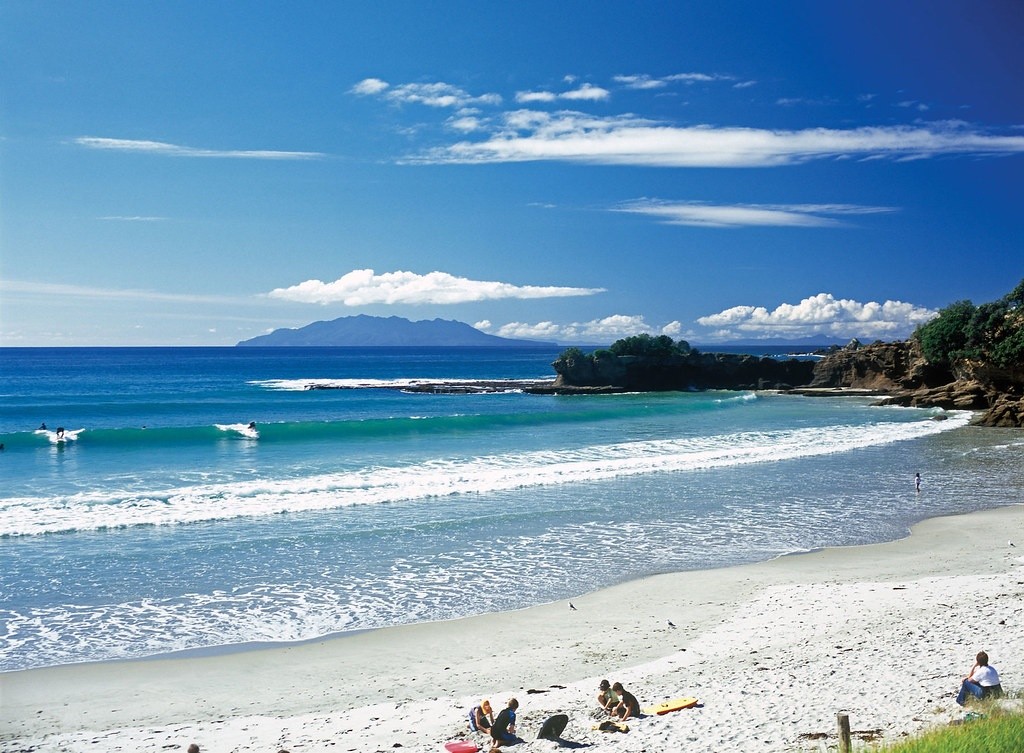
[39,423,46,429]
[57,427,64,437]
[915,473,921,493]
[490,698,519,747]
[956,652,1003,708]
[248,422,255,428]
[469,700,494,734]
[598,680,640,721]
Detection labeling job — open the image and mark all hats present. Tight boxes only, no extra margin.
[481,700,491,714]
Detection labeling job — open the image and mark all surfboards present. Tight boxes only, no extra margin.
[445,740,483,753]
[644,697,698,715]
